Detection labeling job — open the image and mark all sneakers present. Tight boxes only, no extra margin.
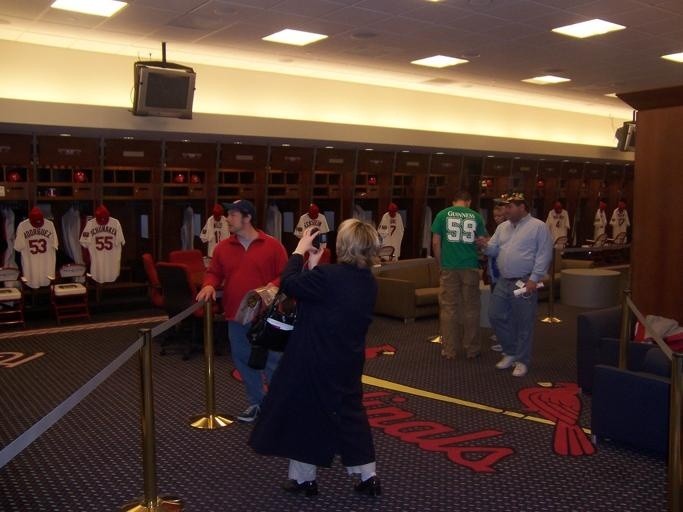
[496,357,519,369]
[487,335,498,341]
[238,403,261,421]
[512,362,527,376]
[492,344,502,351]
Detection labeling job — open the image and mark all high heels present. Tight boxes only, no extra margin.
[355,475,382,495]
[300,482,318,499]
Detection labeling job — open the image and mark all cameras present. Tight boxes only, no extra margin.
[310,228,337,249]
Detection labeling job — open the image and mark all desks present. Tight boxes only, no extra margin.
[561,268,619,307]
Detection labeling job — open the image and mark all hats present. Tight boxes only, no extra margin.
[388,203,399,217]
[369,178,376,185]
[29,208,44,228]
[223,200,255,214]
[174,173,184,181]
[193,176,202,183]
[553,202,562,214]
[212,204,224,221]
[76,173,88,182]
[599,202,604,212]
[6,171,25,183]
[308,204,320,220]
[618,201,625,214]
[95,205,109,225]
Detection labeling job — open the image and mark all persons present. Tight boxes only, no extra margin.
[430,189,490,359]
[195,200,288,423]
[246,219,382,501]
[475,194,553,377]
[482,194,509,353]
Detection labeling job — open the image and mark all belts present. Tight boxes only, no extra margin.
[504,274,530,282]
[491,193,524,206]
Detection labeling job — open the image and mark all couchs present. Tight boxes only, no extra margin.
[578,304,682,392]
[375,259,441,322]
[538,244,593,302]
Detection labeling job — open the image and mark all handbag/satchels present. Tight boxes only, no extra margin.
[254,288,298,353]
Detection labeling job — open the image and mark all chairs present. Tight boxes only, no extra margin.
[168,250,205,279]
[156,262,219,345]
[582,233,608,268]
[0,267,27,331]
[143,253,167,307]
[610,233,626,264]
[52,263,91,325]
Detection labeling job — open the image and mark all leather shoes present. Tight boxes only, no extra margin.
[441,349,458,360]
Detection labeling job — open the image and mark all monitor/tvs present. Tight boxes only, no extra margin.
[133,61,197,120]
[615,121,636,151]
